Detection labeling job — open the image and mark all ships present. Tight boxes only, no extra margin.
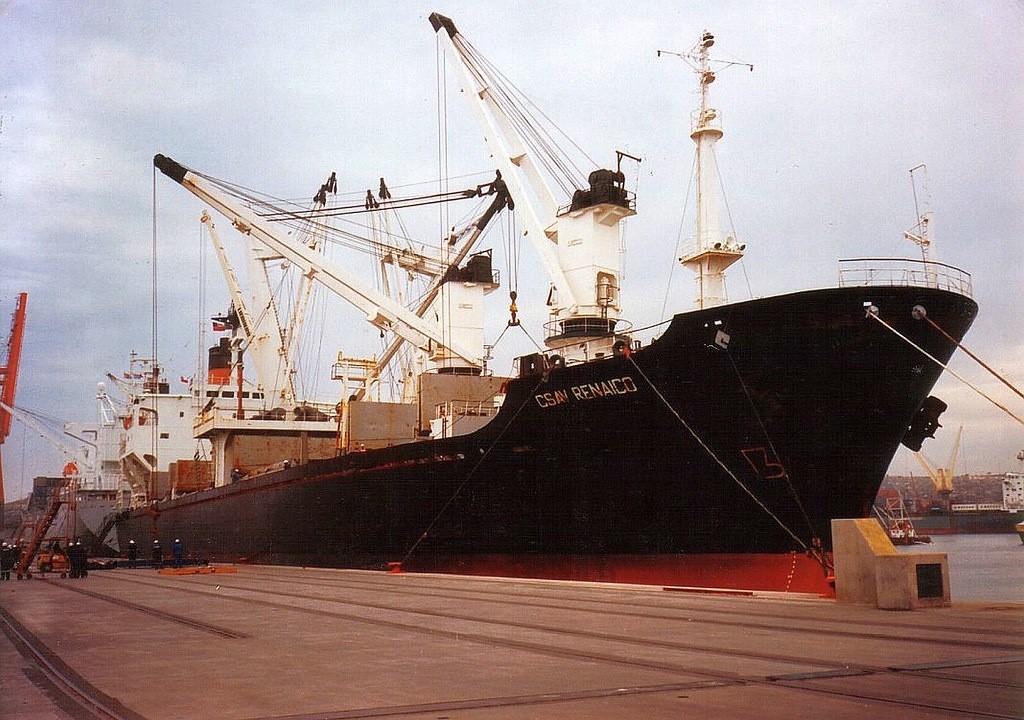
[116,2,981,604]
[42,409,126,556]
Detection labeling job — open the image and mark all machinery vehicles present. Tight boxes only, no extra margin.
[34,543,76,573]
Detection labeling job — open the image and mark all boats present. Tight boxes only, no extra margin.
[879,506,1024,544]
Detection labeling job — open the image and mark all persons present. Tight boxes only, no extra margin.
[232,469,240,484]
[172,539,183,567]
[360,443,366,452]
[153,539,163,571]
[283,460,291,470]
[68,542,87,578]
[0,542,18,580]
[127,540,138,568]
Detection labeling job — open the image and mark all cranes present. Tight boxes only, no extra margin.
[910,424,968,509]
